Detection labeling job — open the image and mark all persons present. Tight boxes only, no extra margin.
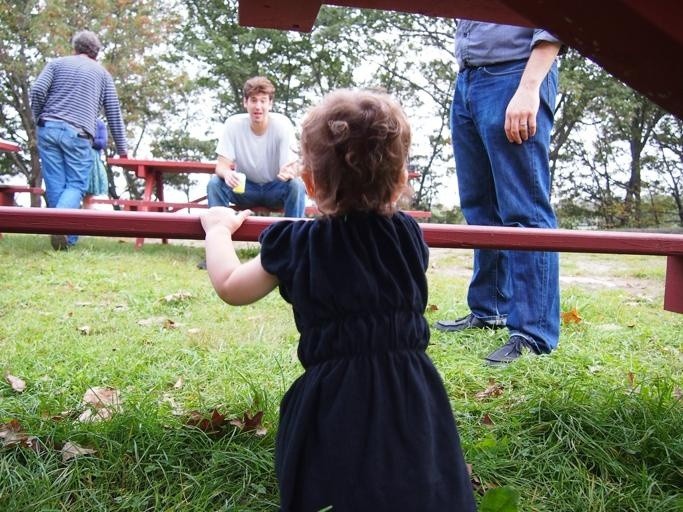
[426,12,568,363]
[195,75,306,271]
[29,29,127,252]
[199,89,479,512]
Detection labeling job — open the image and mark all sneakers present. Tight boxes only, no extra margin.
[50,234,67,251]
[433,314,501,333]
[483,335,547,369]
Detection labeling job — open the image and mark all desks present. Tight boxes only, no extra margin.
[107,158,421,251]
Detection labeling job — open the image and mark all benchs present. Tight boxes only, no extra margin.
[0,186,683,313]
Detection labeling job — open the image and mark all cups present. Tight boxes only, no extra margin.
[233,172,246,195]
